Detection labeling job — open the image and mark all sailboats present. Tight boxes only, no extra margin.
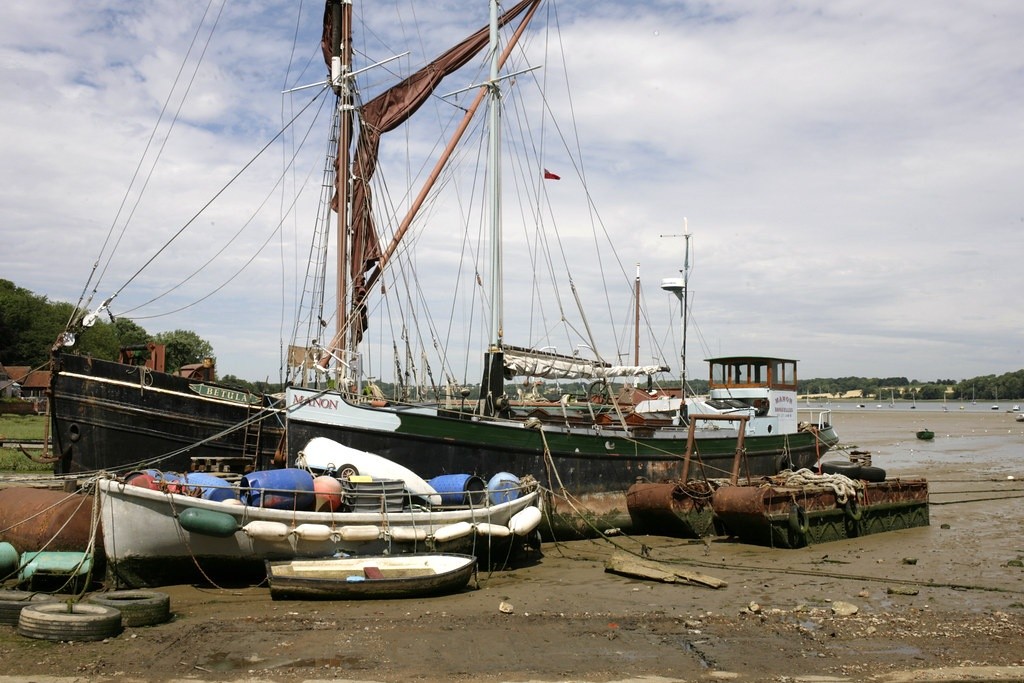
[889,390,895,408]
[911,393,917,410]
[806,387,841,409]
[971,384,976,405]
[991,388,999,410]
[942,393,949,413]
[876,390,882,409]
[44,0,836,499]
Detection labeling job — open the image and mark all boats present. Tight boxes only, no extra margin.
[1013,405,1020,411]
[1016,414,1024,422]
[92,468,543,590]
[856,404,865,408]
[960,406,964,410]
[915,429,935,440]
[264,553,480,602]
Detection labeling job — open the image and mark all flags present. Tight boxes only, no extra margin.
[544,169,560,180]
